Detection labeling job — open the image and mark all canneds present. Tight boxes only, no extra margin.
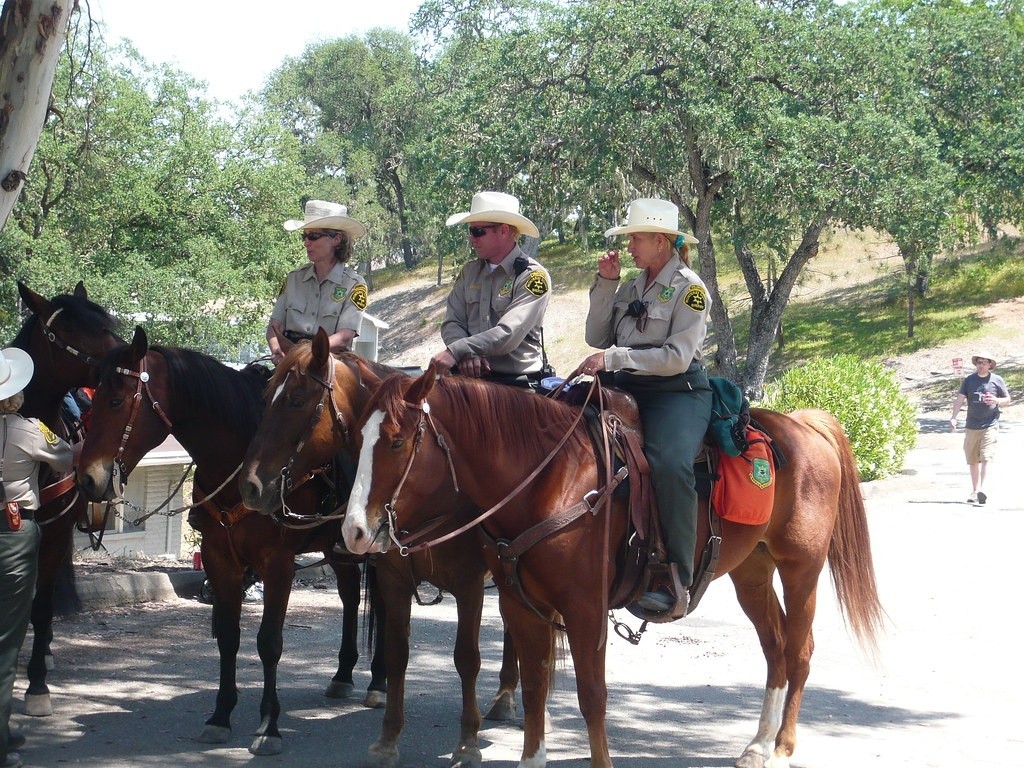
[193,552,203,570]
[985,392,991,405]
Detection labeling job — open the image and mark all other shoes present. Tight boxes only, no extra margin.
[7,731,25,752]
[967,492,978,503]
[334,541,350,556]
[638,584,676,611]
[0,752,23,768]
[977,492,987,504]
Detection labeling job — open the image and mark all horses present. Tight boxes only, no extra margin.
[341,359,889,768]
[238,325,554,768]
[74,325,388,756]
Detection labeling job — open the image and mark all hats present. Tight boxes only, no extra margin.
[446,191,540,238]
[0,348,35,401]
[283,200,366,239]
[605,199,699,244]
[971,352,996,371]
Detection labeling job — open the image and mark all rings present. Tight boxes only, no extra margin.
[588,368,591,370]
[585,367,588,369]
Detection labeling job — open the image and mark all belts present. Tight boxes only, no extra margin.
[0,509,35,521]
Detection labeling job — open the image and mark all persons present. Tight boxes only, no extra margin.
[578,199,712,612]
[267,200,367,366]
[0,347,84,768]
[431,192,551,387]
[949,352,1010,503]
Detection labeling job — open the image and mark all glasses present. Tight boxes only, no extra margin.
[976,361,989,365]
[469,224,511,238]
[301,232,335,242]
